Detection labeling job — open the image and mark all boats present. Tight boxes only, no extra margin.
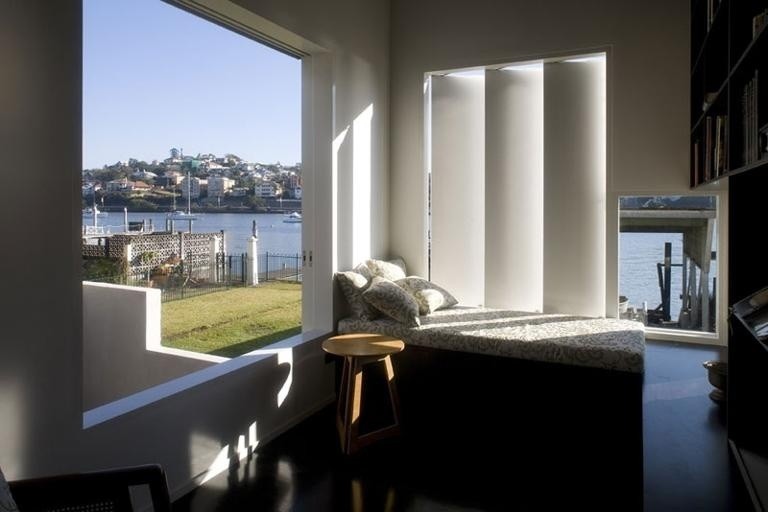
[284,213,301,223]
[83,207,108,217]
[167,210,196,220]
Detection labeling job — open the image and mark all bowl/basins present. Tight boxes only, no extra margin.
[702,360,729,388]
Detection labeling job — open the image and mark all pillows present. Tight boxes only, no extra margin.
[335,257,458,327]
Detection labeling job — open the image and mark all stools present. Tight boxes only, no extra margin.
[321,331,406,455]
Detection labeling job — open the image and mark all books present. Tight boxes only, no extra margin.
[693,68,767,187]
[702,0,721,33]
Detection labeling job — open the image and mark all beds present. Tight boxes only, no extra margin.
[337,302,646,386]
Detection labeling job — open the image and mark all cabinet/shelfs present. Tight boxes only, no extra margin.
[689,1,768,510]
